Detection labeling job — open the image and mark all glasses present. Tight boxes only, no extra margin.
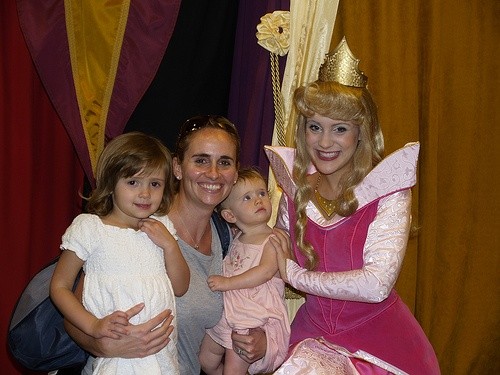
[174,116,239,158]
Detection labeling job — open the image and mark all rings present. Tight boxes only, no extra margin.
[238,348,242,354]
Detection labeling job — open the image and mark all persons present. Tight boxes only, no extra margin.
[65,114,267,375]
[198,167,292,375]
[49,133,190,375]
[269,35,442,375]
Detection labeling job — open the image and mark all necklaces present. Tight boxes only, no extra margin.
[315,174,339,220]
[175,208,206,250]
